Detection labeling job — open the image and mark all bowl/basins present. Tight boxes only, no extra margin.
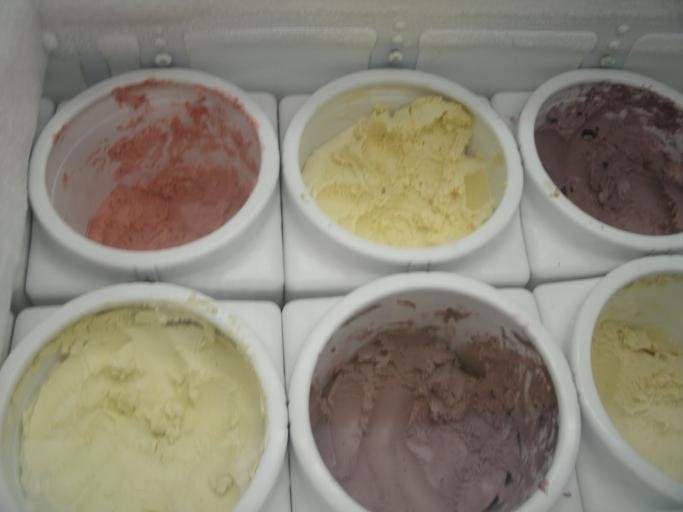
[27,67,280,282]
[570,255,683,506]
[516,68,683,255]
[287,271,583,512]
[280,68,525,270]
[1,281,287,512]
